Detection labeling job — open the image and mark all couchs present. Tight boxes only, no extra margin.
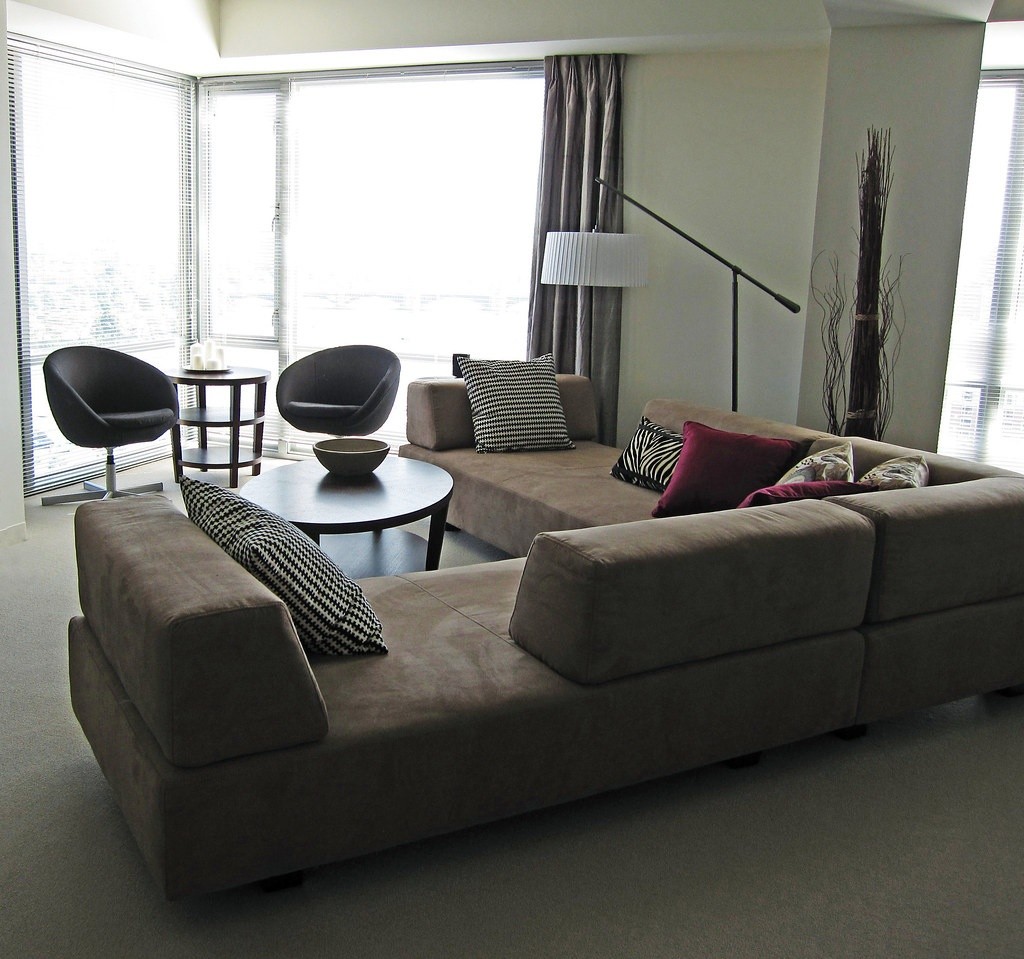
[68,375,1024,901]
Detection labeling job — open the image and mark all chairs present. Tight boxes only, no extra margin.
[276,344,400,439]
[42,346,179,510]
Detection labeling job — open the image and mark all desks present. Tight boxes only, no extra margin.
[166,369,271,488]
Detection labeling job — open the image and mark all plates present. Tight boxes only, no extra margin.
[184,365,231,371]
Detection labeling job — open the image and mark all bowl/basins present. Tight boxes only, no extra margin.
[313,438,390,475]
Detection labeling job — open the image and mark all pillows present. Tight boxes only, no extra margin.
[457,355,575,454]
[736,481,879,509]
[610,416,684,493]
[177,473,390,656]
[774,442,854,485]
[651,422,800,519]
[855,454,929,489]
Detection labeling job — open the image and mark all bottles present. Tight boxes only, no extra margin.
[204,337,214,359]
[190,339,204,369]
[216,343,226,368]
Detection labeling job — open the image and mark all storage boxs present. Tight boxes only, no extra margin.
[453,353,470,376]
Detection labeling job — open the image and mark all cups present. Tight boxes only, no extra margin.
[193,355,202,370]
[206,360,217,369]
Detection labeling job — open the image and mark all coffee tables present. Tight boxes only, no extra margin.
[237,456,453,573]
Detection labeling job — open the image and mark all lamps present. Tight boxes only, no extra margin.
[543,179,800,415]
